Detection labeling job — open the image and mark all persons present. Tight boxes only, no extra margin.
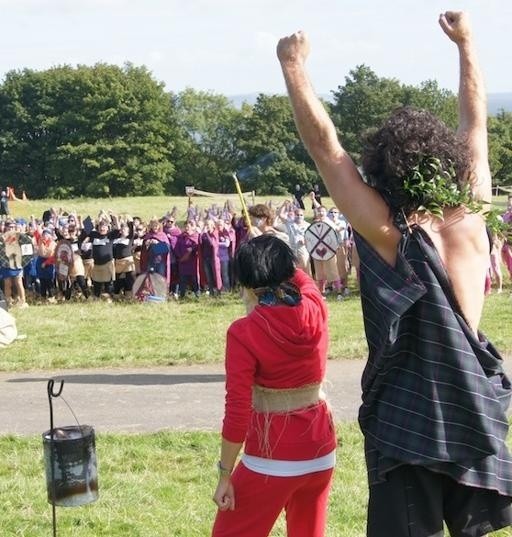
[312,207,345,300]
[285,184,305,210]
[212,227,337,537]
[279,199,312,279]
[484,227,507,296]
[352,240,360,293]
[312,185,321,219]
[502,196,512,280]
[310,192,350,296]
[232,209,249,255]
[287,211,294,222]
[240,204,289,315]
[0,187,235,306]
[277,11,512,537]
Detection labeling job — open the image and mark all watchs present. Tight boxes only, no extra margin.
[217,461,230,478]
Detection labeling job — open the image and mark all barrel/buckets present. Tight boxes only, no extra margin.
[43,393,98,509]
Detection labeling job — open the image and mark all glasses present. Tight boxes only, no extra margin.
[167,221,173,223]
